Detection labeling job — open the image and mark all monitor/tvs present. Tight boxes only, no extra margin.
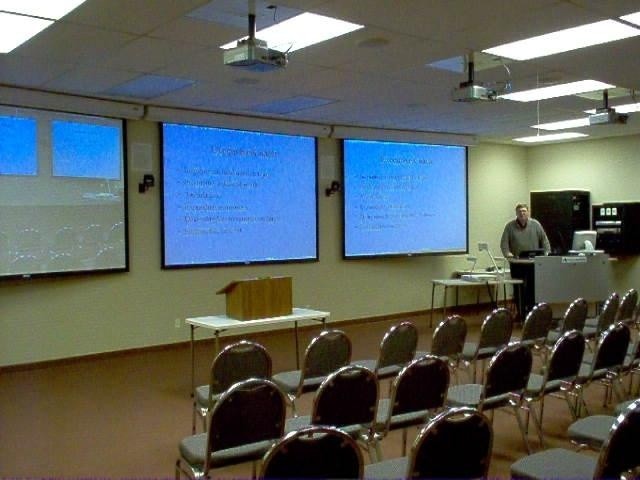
[518,249,545,260]
[568,230,606,257]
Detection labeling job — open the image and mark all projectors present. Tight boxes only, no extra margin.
[452,81,497,103]
[222,38,290,72]
[589,106,628,126]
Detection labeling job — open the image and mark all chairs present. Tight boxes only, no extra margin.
[507,302,552,378]
[545,298,587,370]
[281,365,380,464]
[191,339,274,434]
[370,354,449,464]
[583,331,639,405]
[256,424,365,480]
[577,323,629,416]
[525,329,585,447]
[272,331,352,418]
[446,342,534,456]
[349,321,415,401]
[557,292,619,353]
[364,406,494,480]
[415,315,471,386]
[176,378,286,480]
[462,307,512,386]
[510,400,640,480]
[584,290,638,330]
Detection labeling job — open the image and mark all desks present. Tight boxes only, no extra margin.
[428,278,524,328]
[184,304,329,397]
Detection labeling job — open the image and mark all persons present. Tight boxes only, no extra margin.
[499,204,552,322]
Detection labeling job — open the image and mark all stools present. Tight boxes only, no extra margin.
[567,414,617,460]
